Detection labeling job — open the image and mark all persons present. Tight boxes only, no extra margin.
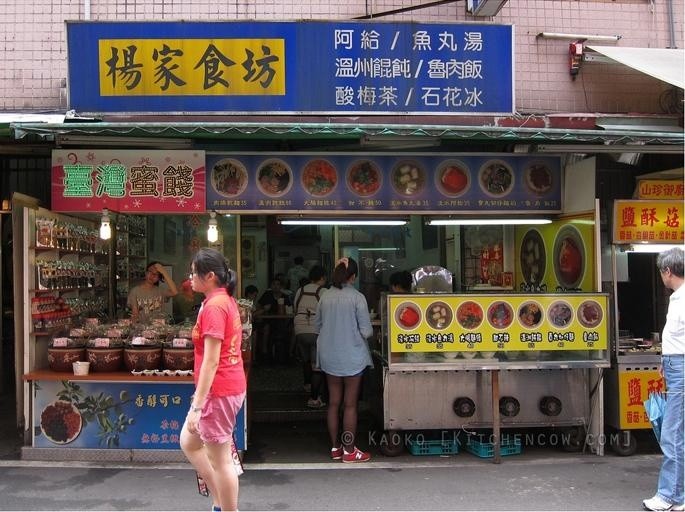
[642,247,685,512]
[315,257,374,463]
[244,285,272,318]
[127,261,178,317]
[293,265,328,408]
[179,247,248,512]
[257,278,291,371]
[389,270,412,294]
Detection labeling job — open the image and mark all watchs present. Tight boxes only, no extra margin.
[189,403,202,413]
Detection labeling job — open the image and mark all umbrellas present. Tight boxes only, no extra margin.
[644,368,667,446]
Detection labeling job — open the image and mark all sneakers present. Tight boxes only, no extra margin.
[330,446,344,461]
[341,447,371,463]
[307,397,326,407]
[642,495,683,511]
[301,383,312,393]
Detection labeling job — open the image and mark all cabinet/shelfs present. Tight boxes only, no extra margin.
[604,331,666,456]
[371,290,611,464]
[21,365,250,461]
[28,213,148,337]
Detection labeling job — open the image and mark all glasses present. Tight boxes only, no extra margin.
[187,271,208,279]
[146,269,161,280]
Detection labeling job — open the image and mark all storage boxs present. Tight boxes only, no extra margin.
[407,438,458,456]
[462,436,522,458]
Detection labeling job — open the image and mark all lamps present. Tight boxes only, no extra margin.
[275,214,411,226]
[423,215,553,226]
[207,212,219,244]
[99,208,112,240]
